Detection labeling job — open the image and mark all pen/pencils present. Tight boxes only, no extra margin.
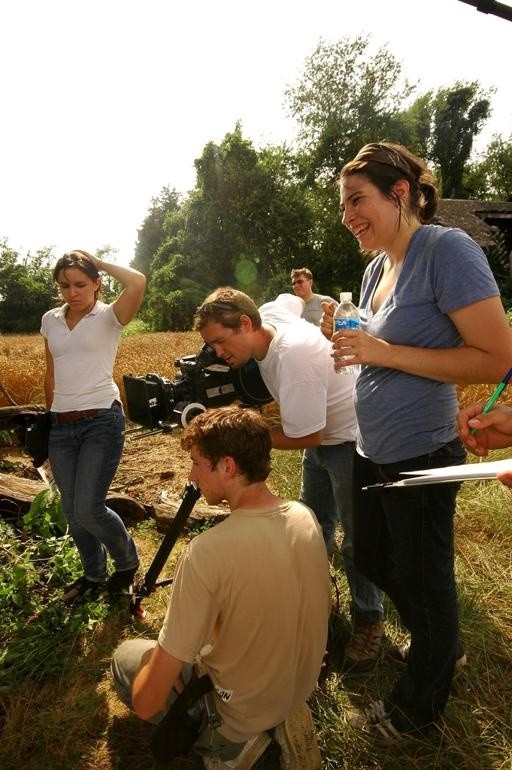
[471,367,512,434]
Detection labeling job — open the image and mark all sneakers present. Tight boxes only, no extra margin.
[274,702,324,769]
[61,576,104,602]
[198,693,272,769]
[341,610,385,674]
[106,562,139,594]
[345,697,432,737]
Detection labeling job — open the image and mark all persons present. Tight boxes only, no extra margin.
[320,141,512,754]
[112,407,333,769]
[193,287,386,666]
[290,269,337,327]
[39,250,142,603]
[456,400,512,488]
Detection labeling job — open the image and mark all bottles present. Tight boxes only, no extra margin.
[333,292,363,377]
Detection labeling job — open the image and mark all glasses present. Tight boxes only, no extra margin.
[290,279,304,286]
